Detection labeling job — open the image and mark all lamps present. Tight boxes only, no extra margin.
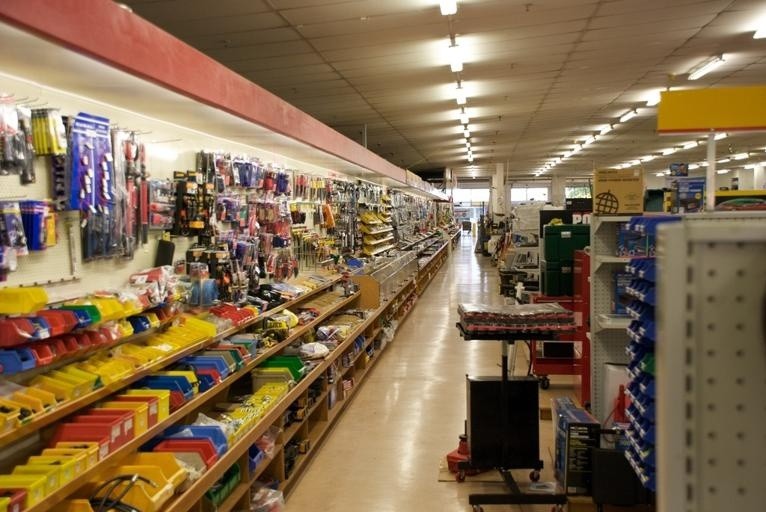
[599,125,613,135]
[447,44,464,73]
[460,113,468,126]
[455,86,467,106]
[585,135,596,146]
[618,108,637,124]
[646,87,670,107]
[687,53,726,80]
[465,142,474,163]
[463,129,470,139]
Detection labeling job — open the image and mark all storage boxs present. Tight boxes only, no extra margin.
[498,270,528,283]
[539,258,575,296]
[549,394,576,475]
[556,408,602,498]
[500,284,518,295]
[543,224,590,261]
[591,167,644,216]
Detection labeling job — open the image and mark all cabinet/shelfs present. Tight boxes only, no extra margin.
[456,321,577,512]
[589,212,765,512]
[0,94,463,512]
[530,254,590,390]
